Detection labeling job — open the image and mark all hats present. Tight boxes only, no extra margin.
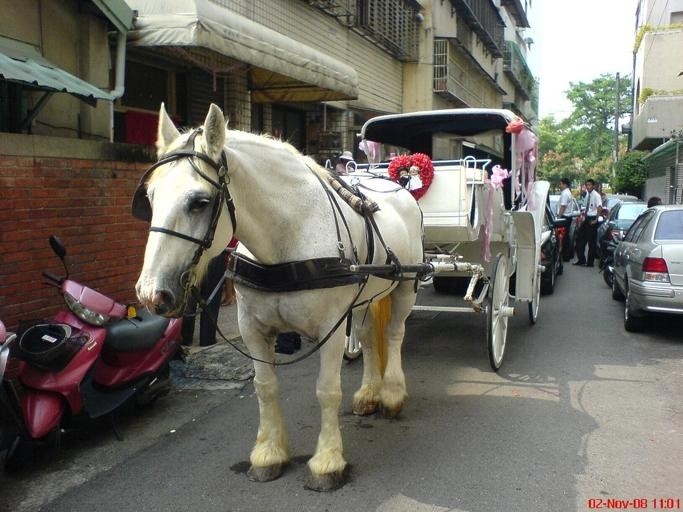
[339,150,354,161]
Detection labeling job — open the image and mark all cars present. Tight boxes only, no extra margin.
[547,190,654,289]
[431,202,563,295]
[608,203,682,332]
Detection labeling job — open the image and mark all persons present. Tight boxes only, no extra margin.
[183,251,236,347]
[556,178,605,267]
[336,151,354,176]
[397,165,421,190]
[647,197,661,206]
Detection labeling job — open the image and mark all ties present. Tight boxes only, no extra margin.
[586,193,590,212]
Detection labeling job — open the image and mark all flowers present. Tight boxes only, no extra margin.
[388,153,435,201]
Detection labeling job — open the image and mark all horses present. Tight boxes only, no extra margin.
[132,101,425,495]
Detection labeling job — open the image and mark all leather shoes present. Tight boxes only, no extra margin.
[572,260,594,267]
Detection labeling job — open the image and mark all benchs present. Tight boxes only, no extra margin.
[375,159,490,185]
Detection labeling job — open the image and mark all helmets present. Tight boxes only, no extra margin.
[19,323,72,364]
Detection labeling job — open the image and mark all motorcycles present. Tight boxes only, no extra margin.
[18,234,191,466]
[0,320,17,387]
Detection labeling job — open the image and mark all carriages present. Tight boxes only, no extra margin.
[130,104,552,494]
